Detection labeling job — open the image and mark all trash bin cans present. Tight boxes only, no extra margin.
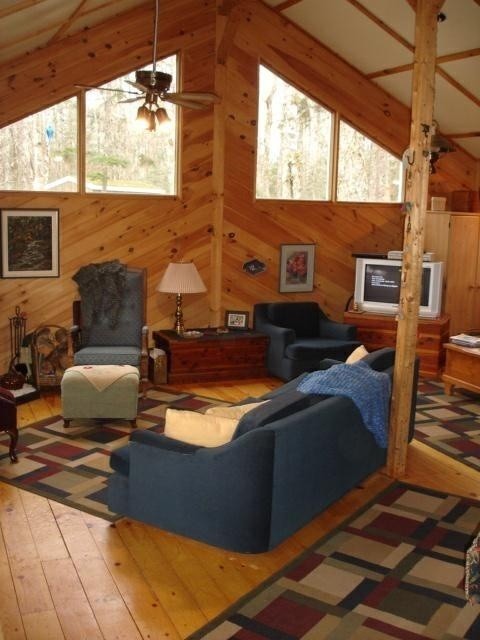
[148,348,168,385]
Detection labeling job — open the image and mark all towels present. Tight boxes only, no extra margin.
[64,365,141,393]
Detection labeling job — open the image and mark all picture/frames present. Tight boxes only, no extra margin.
[224,310,250,330]
[0,208,61,279]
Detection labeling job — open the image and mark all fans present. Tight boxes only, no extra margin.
[74,0,222,111]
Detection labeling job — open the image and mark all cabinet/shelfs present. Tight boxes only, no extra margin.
[444,215,480,337]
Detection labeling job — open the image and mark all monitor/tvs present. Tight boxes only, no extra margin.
[353,257,444,320]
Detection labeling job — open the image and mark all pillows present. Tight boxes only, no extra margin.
[163,344,370,448]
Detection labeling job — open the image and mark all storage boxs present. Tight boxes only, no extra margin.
[451,190,474,213]
[430,196,447,211]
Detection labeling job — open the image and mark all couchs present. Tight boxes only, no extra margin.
[106,347,420,554]
[278,243,316,295]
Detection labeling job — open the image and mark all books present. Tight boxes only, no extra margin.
[450,333,480,348]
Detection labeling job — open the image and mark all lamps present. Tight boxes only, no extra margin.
[428,120,456,174]
[135,92,173,133]
[155,255,208,336]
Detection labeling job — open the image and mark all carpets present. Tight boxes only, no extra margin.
[412,376,480,472]
[182,479,480,639]
[0,381,239,525]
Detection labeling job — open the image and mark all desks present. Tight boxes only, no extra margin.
[152,327,271,386]
[441,342,480,396]
[0,388,60,464]
[342,311,451,380]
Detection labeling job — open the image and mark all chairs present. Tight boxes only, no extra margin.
[69,259,150,401]
[252,301,362,383]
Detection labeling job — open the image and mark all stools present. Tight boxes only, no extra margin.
[60,364,141,428]
